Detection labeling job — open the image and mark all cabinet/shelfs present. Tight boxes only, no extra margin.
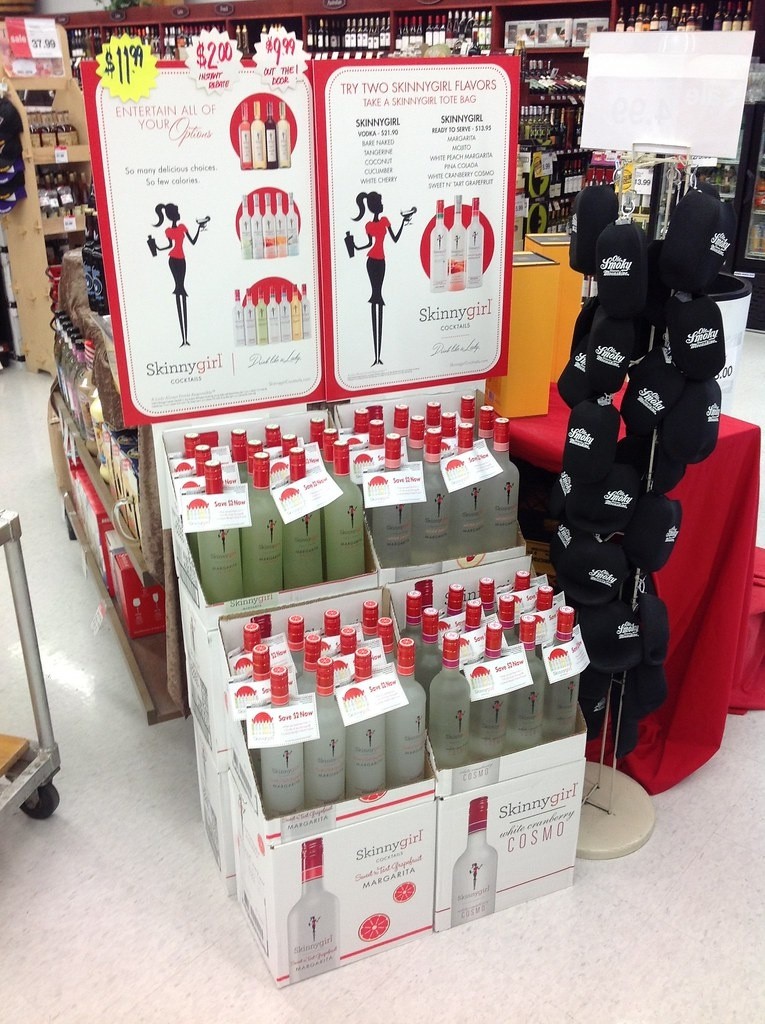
[0,77,102,376]
[0,508,61,823]
[40,0,763,234]
[44,377,182,726]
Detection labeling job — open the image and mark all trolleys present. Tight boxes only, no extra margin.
[0,509,63,828]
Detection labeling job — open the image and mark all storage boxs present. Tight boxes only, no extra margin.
[525,233,584,384]
[503,20,537,48]
[157,384,586,991]
[533,17,573,48]
[572,17,609,48]
[485,249,560,419]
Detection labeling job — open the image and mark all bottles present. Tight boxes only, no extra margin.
[450,796,498,929]
[56,310,112,484]
[184,395,518,600]
[288,838,339,983]
[520,106,584,149]
[627,189,651,216]
[26,110,81,148]
[67,24,282,61]
[34,167,87,211]
[83,174,110,315]
[45,232,71,264]
[615,0,753,32]
[233,101,313,348]
[548,155,591,233]
[308,10,492,57]
[212,573,573,816]
[430,198,484,293]
[748,177,765,252]
[515,43,585,94]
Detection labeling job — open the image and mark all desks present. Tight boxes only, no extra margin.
[491,381,764,786]
